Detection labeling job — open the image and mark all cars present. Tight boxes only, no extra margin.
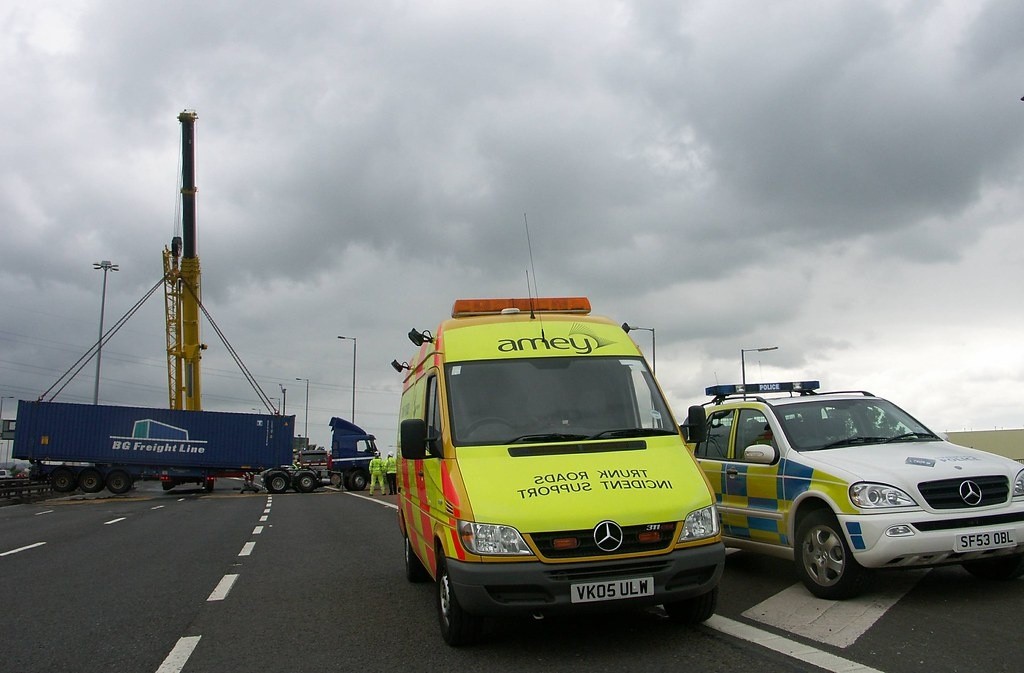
[682,380,1023,601]
[0,470,27,479]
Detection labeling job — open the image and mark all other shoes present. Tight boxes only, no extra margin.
[384,493,386,495]
[395,493,398,494]
[370,494,373,496]
[389,493,393,494]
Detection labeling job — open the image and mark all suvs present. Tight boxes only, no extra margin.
[296,449,328,467]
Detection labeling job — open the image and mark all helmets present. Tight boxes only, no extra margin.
[388,451,394,456]
[374,451,380,456]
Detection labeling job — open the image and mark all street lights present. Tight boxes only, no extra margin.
[629,326,655,376]
[296,378,309,448]
[252,408,261,414]
[0,396,15,437]
[270,397,281,414]
[338,336,356,424]
[742,347,779,401]
[93,260,119,406]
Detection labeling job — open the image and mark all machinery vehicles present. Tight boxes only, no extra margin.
[159,110,216,492]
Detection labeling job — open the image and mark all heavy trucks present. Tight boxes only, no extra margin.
[12,400,382,495]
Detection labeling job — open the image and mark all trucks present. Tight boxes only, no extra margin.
[293,436,309,459]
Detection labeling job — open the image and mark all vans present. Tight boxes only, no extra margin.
[391,298,726,650]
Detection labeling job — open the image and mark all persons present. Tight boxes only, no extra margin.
[384,451,396,495]
[369,451,386,496]
